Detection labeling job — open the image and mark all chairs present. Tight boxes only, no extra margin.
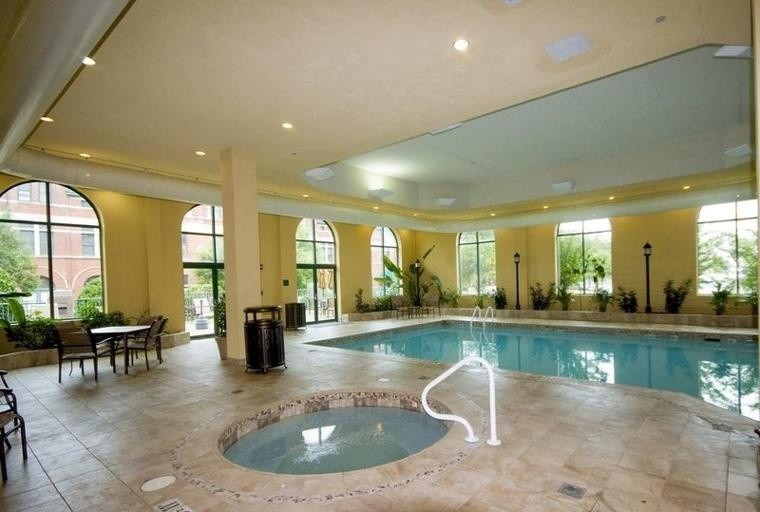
[0,312,169,480]
[325,298,335,318]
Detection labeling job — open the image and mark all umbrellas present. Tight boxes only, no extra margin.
[318,268,334,297]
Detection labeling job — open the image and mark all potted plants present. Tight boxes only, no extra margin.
[213,292,227,361]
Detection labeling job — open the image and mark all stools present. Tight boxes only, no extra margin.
[397,305,440,320]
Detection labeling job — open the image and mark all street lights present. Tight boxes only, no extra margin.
[640,241,652,312]
[513,251,521,309]
[413,260,423,306]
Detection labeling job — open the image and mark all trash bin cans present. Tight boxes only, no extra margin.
[243,305,288,375]
[284,302,306,331]
